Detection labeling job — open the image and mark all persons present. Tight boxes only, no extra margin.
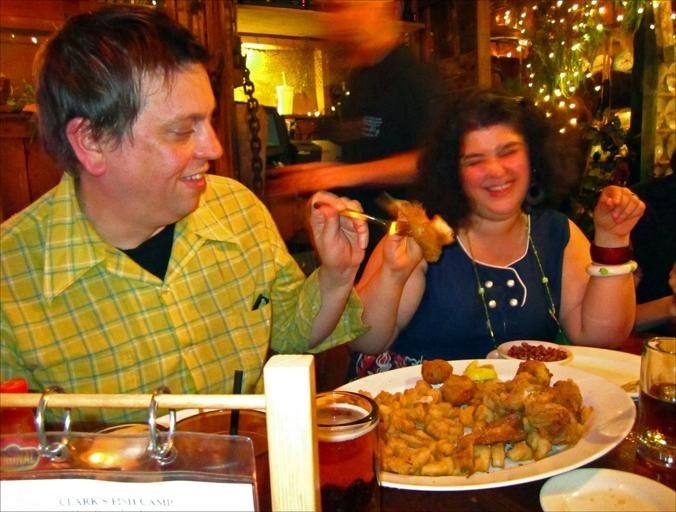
[626,147,676,338]
[348,86,646,383]
[0,3,370,424]
[261,0,451,286]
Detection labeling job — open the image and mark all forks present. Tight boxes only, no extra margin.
[312,202,414,238]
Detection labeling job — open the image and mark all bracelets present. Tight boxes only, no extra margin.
[590,239,633,265]
[585,260,638,277]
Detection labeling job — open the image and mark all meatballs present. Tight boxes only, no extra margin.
[421,359,453,384]
[554,379,582,413]
[441,375,476,407]
[516,359,554,388]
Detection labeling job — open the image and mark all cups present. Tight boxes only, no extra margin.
[640,337,676,421]
[314,389,386,511]
[149,409,270,511]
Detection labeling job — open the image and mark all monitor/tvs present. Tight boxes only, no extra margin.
[252,106,292,168]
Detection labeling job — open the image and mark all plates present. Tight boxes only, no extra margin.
[486,343,643,401]
[329,358,637,492]
[539,467,676,512]
[498,340,575,365]
